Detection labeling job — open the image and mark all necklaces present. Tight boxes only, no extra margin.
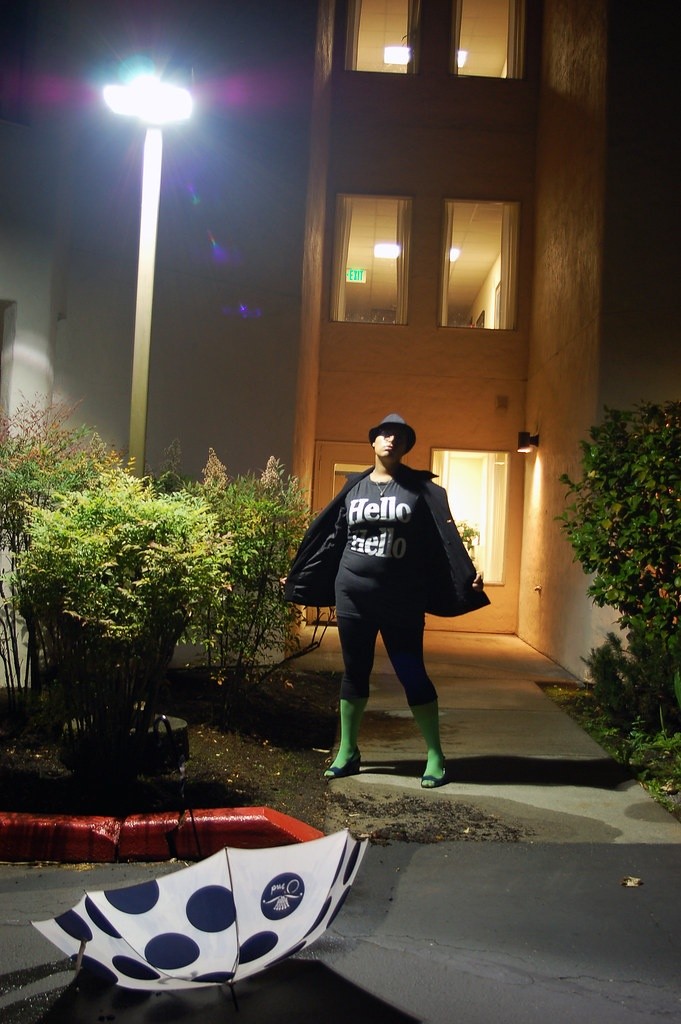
[372,472,393,496]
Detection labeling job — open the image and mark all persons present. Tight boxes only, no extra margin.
[279,412,491,788]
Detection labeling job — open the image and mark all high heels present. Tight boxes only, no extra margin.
[324,746,362,778]
[422,756,447,788]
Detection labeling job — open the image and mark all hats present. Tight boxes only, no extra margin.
[369,414,416,455]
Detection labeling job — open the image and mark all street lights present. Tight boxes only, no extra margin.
[97,73,200,477]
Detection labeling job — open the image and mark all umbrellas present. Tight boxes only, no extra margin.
[28,826,369,994]
